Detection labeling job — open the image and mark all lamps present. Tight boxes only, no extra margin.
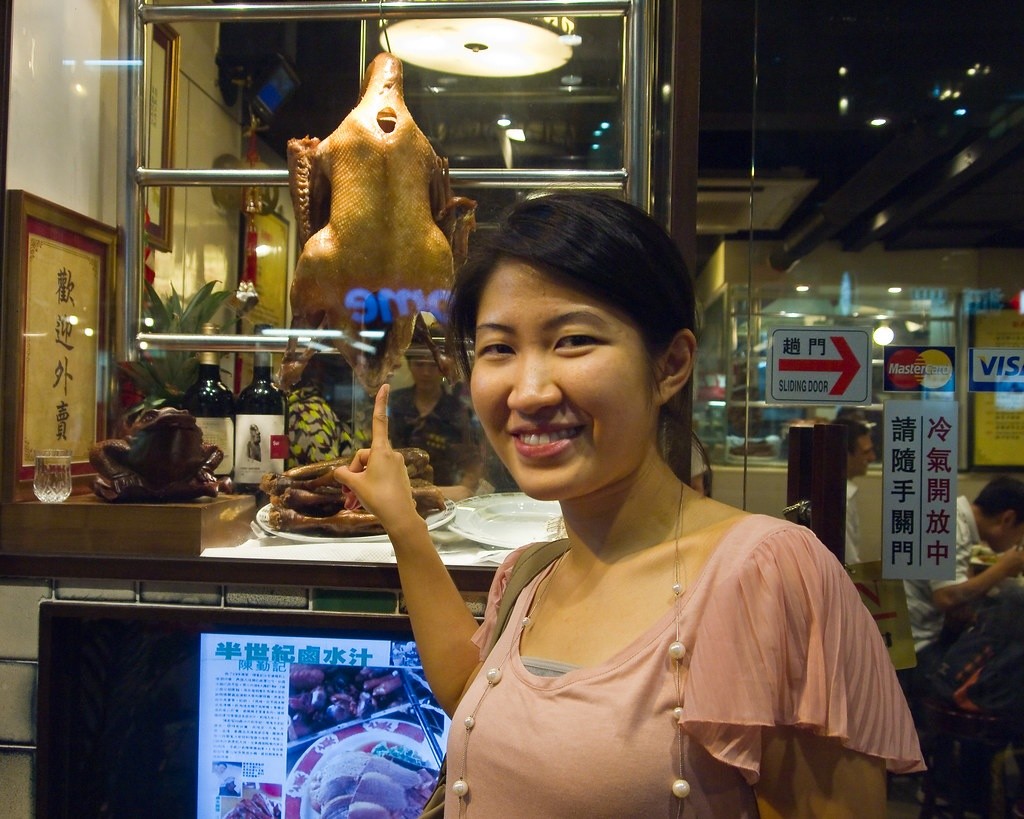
[378,9,578,80]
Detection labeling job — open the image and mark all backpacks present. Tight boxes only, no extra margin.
[925,586,1024,717]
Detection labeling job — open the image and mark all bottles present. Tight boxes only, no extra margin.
[232,323,289,489]
[179,322,235,484]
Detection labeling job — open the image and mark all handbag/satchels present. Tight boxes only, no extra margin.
[420,538,571,818]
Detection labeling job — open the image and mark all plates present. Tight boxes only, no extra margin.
[254,494,456,545]
[442,492,573,551]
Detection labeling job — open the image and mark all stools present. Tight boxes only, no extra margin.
[920,695,1024,819]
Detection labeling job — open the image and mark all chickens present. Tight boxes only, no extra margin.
[264,444,445,535]
[277,53,477,398]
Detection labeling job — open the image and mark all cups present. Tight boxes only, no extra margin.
[32,456,73,503]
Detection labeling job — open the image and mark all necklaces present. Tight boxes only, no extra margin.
[452,483,691,819]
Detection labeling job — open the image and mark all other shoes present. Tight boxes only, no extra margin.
[915,776,989,808]
[1012,799,1024,819]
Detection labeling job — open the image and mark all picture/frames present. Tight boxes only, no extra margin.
[2,188,122,504]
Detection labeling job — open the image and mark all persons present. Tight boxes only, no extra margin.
[332,187,928,819]
[384,335,485,500]
[690,444,705,495]
[829,419,873,568]
[892,477,1024,807]
